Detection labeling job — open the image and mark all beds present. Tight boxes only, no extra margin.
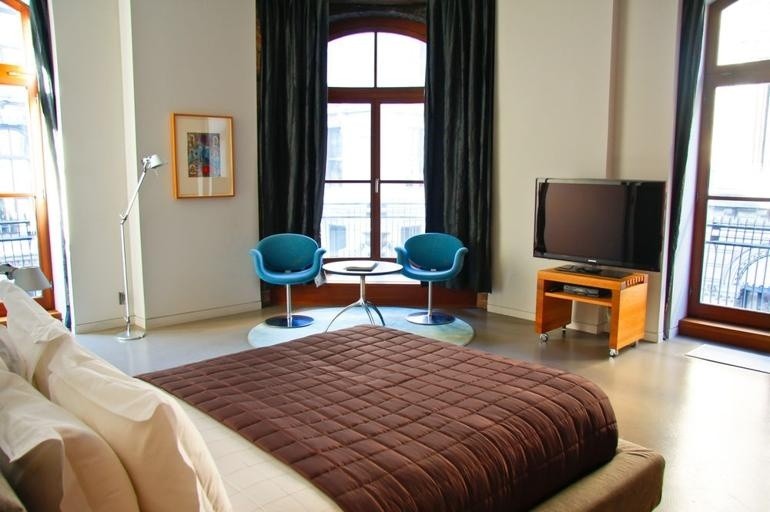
[1,257,667,510]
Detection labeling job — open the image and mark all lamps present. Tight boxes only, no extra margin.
[116,153,163,342]
[0,259,51,294]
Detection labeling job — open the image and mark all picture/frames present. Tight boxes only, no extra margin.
[170,112,235,199]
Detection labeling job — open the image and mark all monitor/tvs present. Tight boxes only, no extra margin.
[532,178,667,279]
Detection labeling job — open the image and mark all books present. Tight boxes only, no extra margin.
[346,261,377,271]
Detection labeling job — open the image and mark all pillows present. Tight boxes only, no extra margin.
[4,287,230,509]
[0,362,139,507]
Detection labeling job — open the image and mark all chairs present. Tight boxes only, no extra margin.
[394,233,469,326]
[249,233,327,329]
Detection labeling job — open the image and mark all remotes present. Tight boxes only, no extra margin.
[575,266,602,273]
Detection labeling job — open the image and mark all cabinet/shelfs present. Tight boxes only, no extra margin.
[533,264,650,356]
